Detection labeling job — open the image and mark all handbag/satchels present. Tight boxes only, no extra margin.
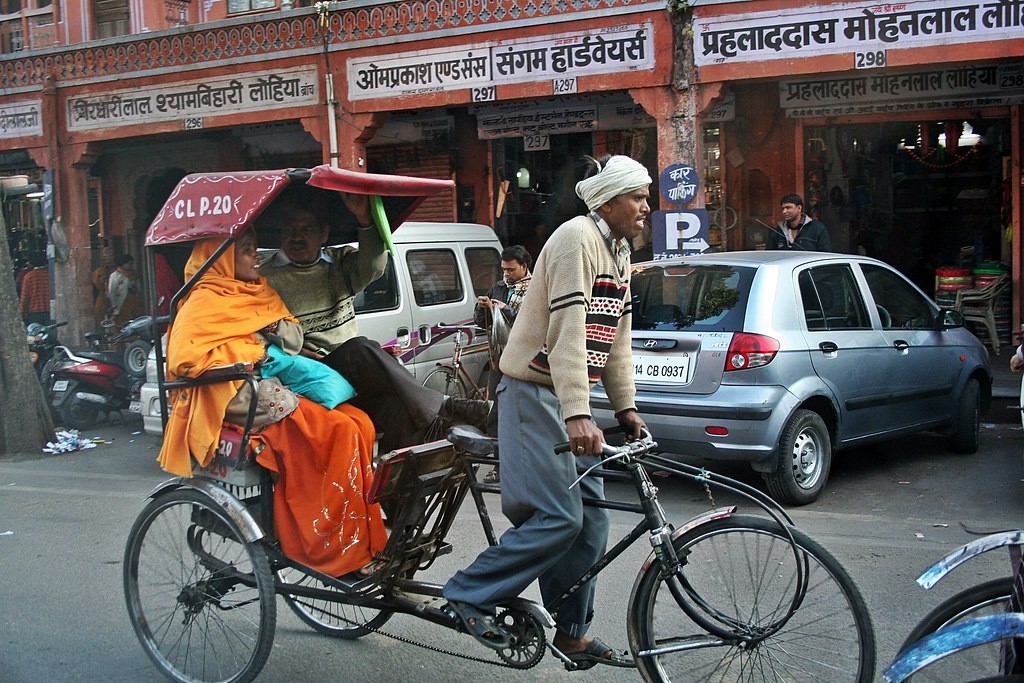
[259,344,359,410]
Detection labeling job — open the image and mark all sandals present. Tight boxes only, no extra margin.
[560,636,637,667]
[351,559,391,581]
[447,595,510,651]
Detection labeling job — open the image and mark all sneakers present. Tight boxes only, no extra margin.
[448,393,495,427]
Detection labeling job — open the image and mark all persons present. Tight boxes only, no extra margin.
[765,193,833,253]
[257,190,499,457]
[522,220,552,275]
[92,267,114,333]
[107,255,143,350]
[19,250,57,338]
[442,153,654,669]
[155,221,387,577]
[473,244,534,485]
[147,252,183,336]
[1010,342,1024,374]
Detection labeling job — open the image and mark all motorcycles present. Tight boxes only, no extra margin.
[15,293,172,437]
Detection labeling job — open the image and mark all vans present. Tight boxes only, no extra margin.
[316,217,511,403]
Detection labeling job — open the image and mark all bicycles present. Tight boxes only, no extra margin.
[885,523,1024,683]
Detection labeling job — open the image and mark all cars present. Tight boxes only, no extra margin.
[589,249,992,506]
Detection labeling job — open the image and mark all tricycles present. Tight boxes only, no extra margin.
[120,168,876,683]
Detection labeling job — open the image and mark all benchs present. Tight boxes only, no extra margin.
[144,240,413,540]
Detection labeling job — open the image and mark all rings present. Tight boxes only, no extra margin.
[577,444,585,451]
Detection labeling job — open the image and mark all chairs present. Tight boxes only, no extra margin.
[953,273,1011,355]
